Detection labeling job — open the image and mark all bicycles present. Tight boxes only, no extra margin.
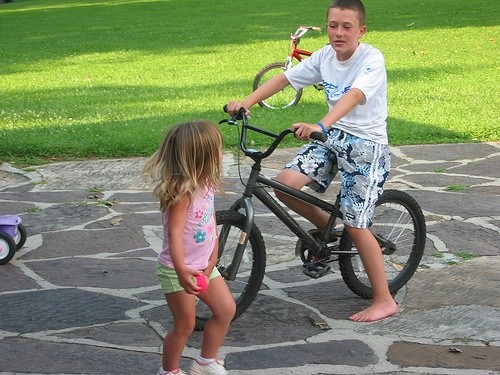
[192,105,427,332]
[251,24,324,111]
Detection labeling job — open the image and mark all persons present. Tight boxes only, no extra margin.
[144,120,236,375]
[226,0,398,322]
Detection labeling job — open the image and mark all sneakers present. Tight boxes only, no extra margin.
[187,359,229,375]
[157,367,187,375]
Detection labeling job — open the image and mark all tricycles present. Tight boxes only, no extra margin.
[0,214,28,266]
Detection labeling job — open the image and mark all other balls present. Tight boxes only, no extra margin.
[191,273,208,293]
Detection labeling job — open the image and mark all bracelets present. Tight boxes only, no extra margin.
[316,122,326,133]
